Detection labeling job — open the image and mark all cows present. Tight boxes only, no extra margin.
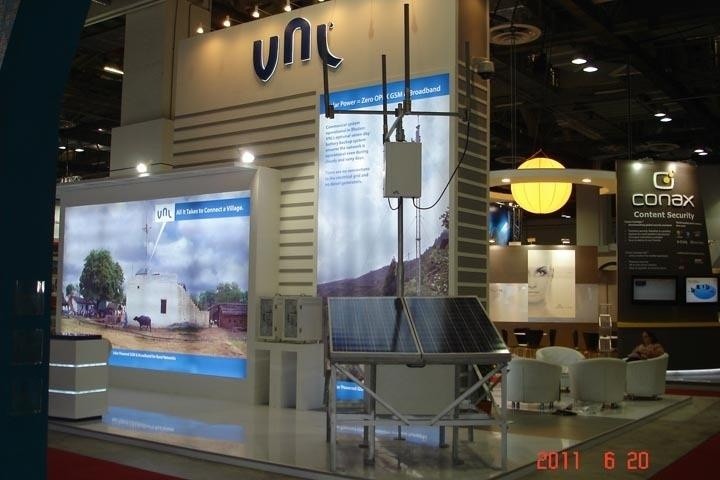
[133,315,152,333]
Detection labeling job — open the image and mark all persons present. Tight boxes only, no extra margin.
[528,249,555,318]
[626,329,663,363]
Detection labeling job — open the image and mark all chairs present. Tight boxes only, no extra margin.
[506,346,670,414]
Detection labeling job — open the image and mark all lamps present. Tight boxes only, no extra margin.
[58,138,86,153]
[508,148,577,217]
[194,0,296,34]
